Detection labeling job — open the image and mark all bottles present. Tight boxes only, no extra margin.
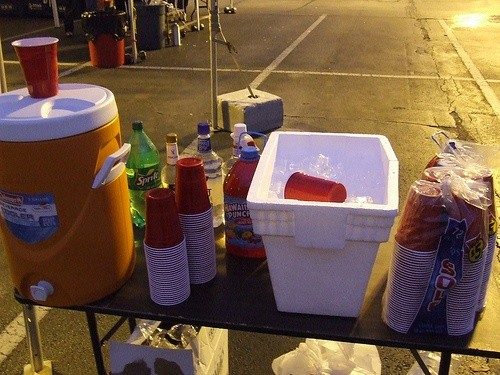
[222,123,248,186]
[160,131,180,194]
[173,23,181,46]
[190,122,222,227]
[124,122,160,229]
[223,132,267,257]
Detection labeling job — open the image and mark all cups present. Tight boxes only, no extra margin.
[284,170,347,203]
[143,158,217,305]
[380,143,496,336]
[11,36,59,99]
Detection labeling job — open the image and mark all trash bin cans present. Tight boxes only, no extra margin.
[133,5,167,50]
[80,10,128,68]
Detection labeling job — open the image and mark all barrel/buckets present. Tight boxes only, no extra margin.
[0,83,136,307]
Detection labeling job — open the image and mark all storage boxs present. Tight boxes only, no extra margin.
[109,319,229,375]
[246,131,399,317]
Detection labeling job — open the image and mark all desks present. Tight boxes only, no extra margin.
[14,215,500,375]
